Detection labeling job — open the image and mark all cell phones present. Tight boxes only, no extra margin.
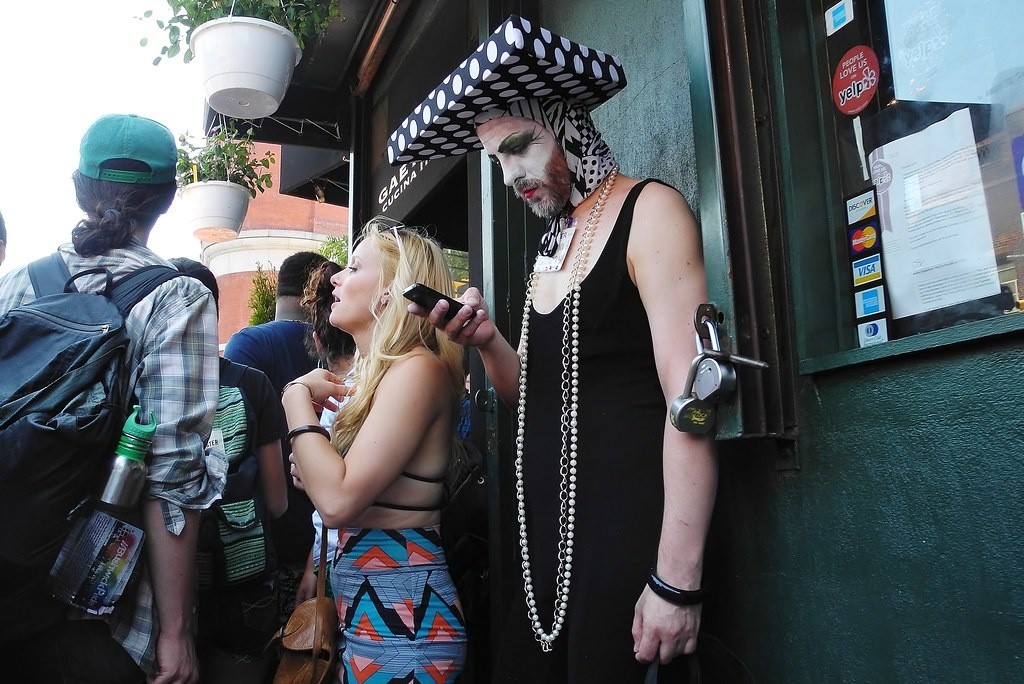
[402,283,478,322]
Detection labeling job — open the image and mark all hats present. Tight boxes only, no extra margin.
[77,113,178,185]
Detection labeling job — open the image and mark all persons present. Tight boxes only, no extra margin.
[0,113,219,684]
[223,252,356,569]
[282,214,466,684]
[0,211,7,268]
[167,257,290,684]
[414,116,755,683]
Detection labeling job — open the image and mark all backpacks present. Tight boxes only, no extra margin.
[197,362,279,592]
[0,252,185,644]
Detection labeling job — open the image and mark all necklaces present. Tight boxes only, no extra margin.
[515,164,620,651]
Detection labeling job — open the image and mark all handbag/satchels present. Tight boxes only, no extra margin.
[442,439,489,507]
[261,597,339,684]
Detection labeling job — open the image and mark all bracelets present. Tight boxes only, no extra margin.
[281,380,313,398]
[286,425,331,447]
[646,568,705,607]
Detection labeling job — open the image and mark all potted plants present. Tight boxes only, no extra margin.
[138,0,347,119]
[176,113,276,243]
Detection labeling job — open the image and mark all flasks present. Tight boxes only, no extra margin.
[99,405,157,505]
